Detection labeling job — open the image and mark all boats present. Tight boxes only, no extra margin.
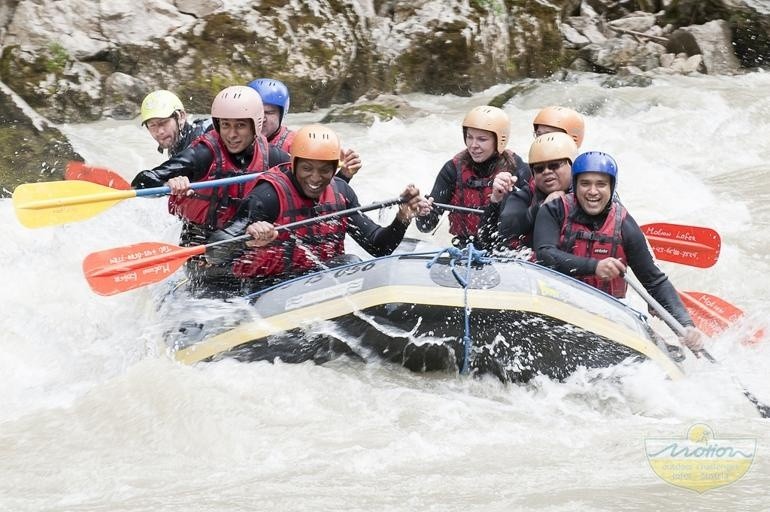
[162,245,687,388]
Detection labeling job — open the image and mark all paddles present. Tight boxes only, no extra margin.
[12,149,357,229]
[83,191,414,296]
[423,194,721,268]
[65,159,133,190]
[676,289,765,349]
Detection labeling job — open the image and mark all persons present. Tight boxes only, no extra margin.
[473,106,585,251]
[249,76,362,182]
[140,89,214,158]
[414,106,531,250]
[130,87,291,245]
[497,131,621,265]
[204,121,418,287]
[533,150,704,354]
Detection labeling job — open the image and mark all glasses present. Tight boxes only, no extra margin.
[534,163,565,173]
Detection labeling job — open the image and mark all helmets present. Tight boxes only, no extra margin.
[248,79,289,128]
[211,86,264,136]
[462,106,508,154]
[533,105,584,147]
[528,132,577,178]
[292,125,339,178]
[571,152,617,193]
[140,90,184,125]
